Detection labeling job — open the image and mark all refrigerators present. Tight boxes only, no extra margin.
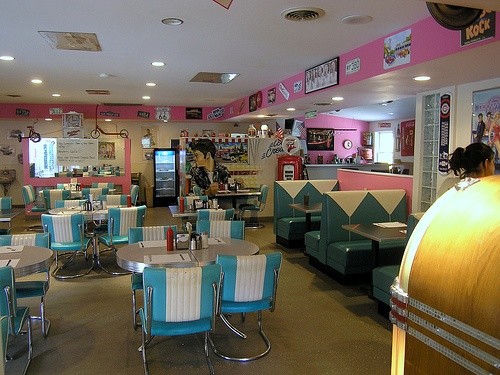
[153,148,179,208]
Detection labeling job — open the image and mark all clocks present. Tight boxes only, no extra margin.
[343,140,353,150]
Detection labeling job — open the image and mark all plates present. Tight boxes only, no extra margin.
[237,190,250,193]
[217,191,231,194]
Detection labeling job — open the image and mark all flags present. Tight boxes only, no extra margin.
[297,122,306,139]
[276,122,283,137]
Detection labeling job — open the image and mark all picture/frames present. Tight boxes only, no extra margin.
[470,87,500,163]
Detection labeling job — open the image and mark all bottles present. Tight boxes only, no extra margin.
[332,153,360,164]
[317,154,323,164]
[76,184,81,191]
[190,232,209,250]
[81,199,107,211]
[190,198,218,211]
[247,124,272,138]
[180,128,188,137]
[304,153,311,164]
[219,177,244,190]
[167,227,173,251]
[127,194,131,207]
[180,197,184,213]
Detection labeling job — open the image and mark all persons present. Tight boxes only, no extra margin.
[448,142,495,178]
[472,112,492,142]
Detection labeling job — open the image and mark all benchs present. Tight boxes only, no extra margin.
[373,212,426,318]
[274,179,339,250]
[324,190,408,281]
[304,188,388,272]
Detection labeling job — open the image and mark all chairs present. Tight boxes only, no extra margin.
[0,181,284,375]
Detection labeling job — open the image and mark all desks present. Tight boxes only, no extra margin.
[168,205,198,233]
[114,238,259,328]
[290,203,322,257]
[343,223,406,300]
[0,245,54,337]
[0,213,19,229]
[216,188,261,210]
[49,205,130,268]
[38,188,116,207]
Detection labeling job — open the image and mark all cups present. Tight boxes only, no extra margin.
[304,192,309,206]
